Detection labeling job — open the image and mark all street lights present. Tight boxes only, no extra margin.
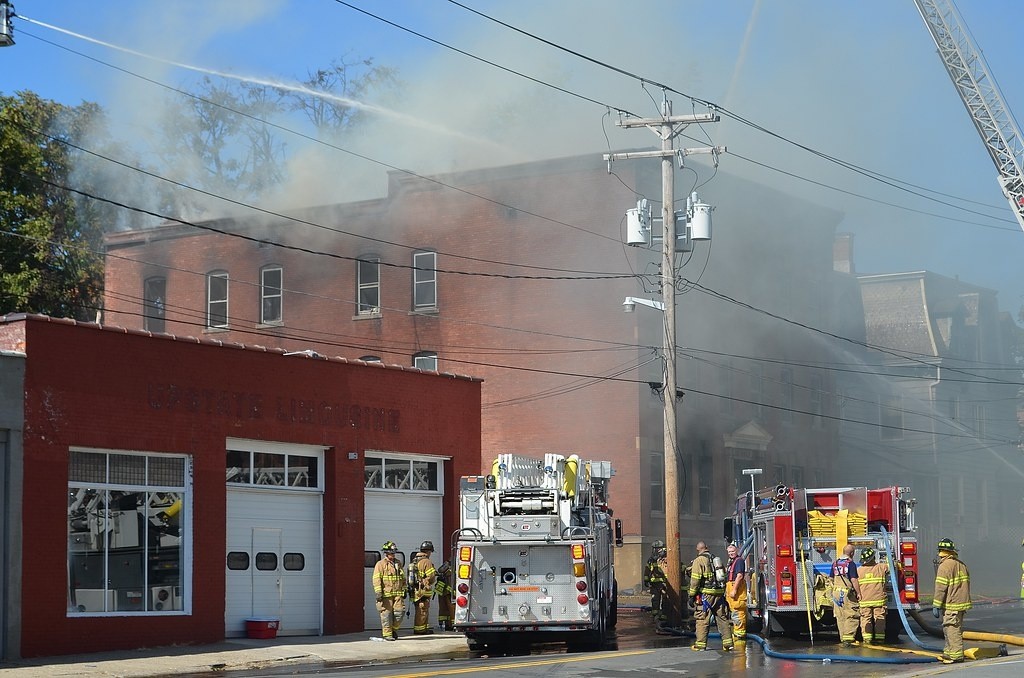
[622,297,684,627]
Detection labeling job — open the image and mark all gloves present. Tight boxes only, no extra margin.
[644,582,649,587]
[932,607,939,618]
[401,592,407,599]
[689,596,695,608]
[376,597,382,603]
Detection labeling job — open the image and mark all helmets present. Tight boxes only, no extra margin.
[859,547,876,565]
[420,541,434,553]
[658,548,667,557]
[937,537,956,551]
[381,540,398,554]
[651,539,663,548]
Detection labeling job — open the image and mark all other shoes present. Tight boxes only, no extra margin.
[722,644,734,650]
[942,657,964,664]
[863,638,873,643]
[445,626,454,631]
[384,636,395,641]
[937,653,950,660]
[414,629,433,634]
[877,639,885,643]
[843,640,859,645]
[731,633,747,645]
[691,644,706,652]
[392,631,398,639]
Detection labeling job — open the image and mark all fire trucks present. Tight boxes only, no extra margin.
[723,468,923,640]
[450,452,624,653]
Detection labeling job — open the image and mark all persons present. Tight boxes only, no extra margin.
[725,544,748,640]
[409,540,434,634]
[857,546,898,644]
[932,537,973,664]
[373,540,407,641]
[688,540,734,651]
[644,541,687,622]
[828,545,862,646]
